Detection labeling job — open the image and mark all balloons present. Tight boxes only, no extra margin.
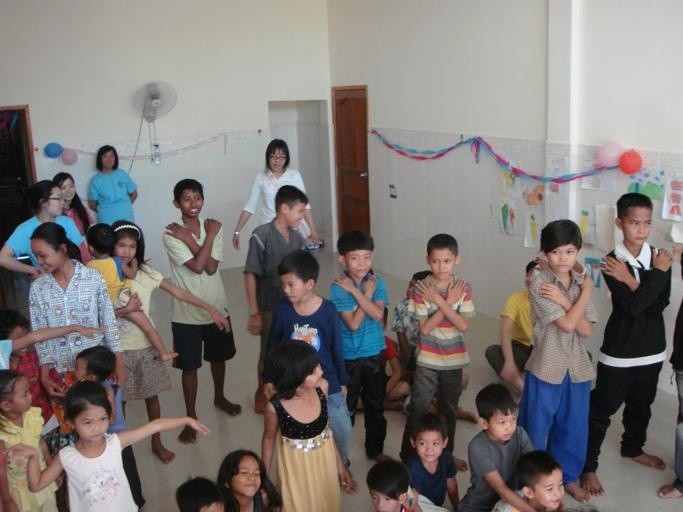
[44,143,63,158]
[618,149,641,175]
[596,142,625,167]
[61,147,77,165]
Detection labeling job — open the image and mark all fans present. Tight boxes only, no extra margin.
[133,79,179,166]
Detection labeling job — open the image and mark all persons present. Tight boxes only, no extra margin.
[85,144,138,226]
[0,173,597,512]
[656,247,682,500]
[232,140,320,250]
[579,191,672,497]
